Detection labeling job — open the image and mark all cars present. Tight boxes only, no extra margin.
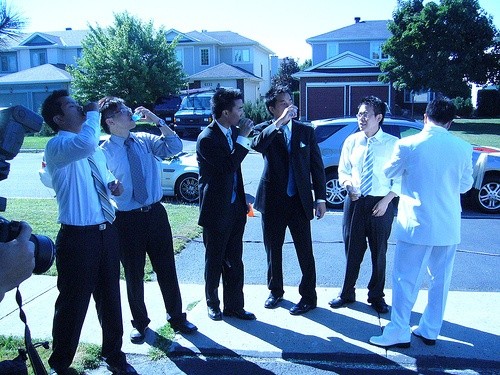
[154,98,182,126]
[37,135,204,204]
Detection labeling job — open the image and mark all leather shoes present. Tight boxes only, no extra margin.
[367,294,389,313]
[411,326,437,346]
[207,305,222,320]
[327,295,356,307]
[264,290,284,309]
[368,334,410,348]
[223,306,256,320]
[129,322,149,344]
[288,296,317,315]
[170,319,198,334]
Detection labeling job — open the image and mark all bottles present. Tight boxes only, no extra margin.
[248,203,254,216]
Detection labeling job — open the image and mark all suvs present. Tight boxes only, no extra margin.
[174,94,217,134]
[301,119,500,211]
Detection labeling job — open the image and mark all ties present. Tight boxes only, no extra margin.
[225,130,238,205]
[281,125,297,196]
[87,155,116,225]
[123,138,149,205]
[359,138,375,198]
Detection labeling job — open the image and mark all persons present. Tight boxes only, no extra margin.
[43,90,138,375]
[370,100,474,348]
[250,85,327,315]
[0,221,36,304]
[196,89,255,319]
[328,95,403,313]
[98,96,198,342]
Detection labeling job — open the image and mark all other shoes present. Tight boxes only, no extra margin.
[101,350,126,368]
[49,365,66,375]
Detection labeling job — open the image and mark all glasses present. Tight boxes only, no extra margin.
[356,114,375,118]
[106,107,132,118]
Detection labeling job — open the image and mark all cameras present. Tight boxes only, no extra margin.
[0,216,57,276]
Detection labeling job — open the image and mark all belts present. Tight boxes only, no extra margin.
[117,201,163,213]
[60,222,112,231]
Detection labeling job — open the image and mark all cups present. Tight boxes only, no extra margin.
[288,107,297,117]
[132,112,141,121]
[352,187,360,198]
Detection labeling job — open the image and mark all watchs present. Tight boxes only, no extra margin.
[156,119,165,127]
